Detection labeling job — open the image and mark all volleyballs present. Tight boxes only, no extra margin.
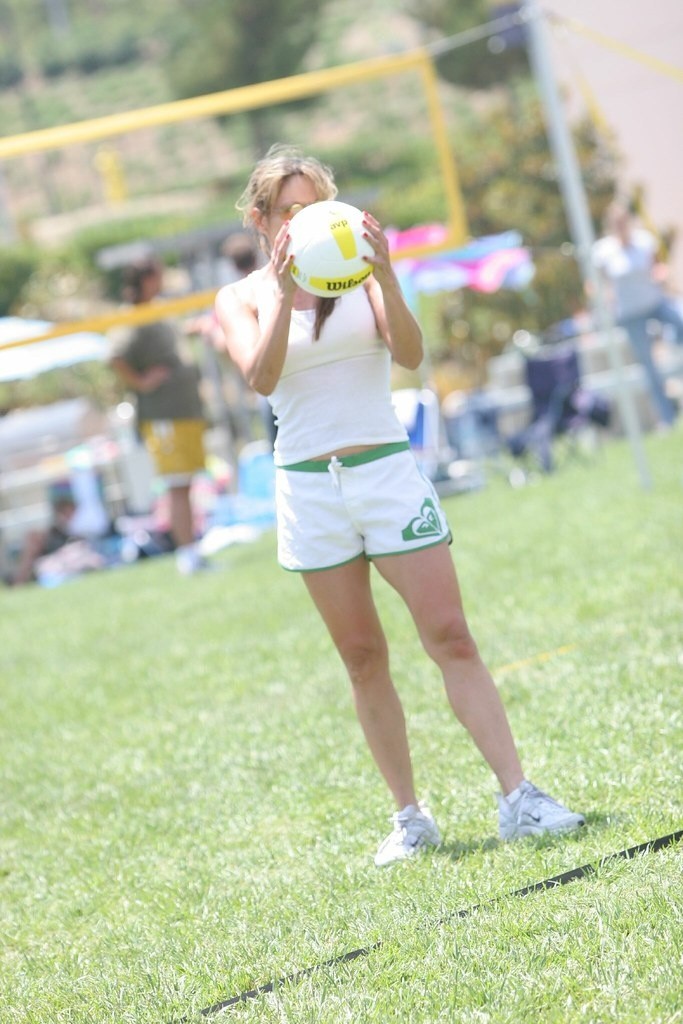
[283,200,380,301]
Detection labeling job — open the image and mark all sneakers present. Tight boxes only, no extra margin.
[374,801,439,867]
[496,781,585,841]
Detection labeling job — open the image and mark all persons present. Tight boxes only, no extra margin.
[212,150,586,870]
[585,195,683,431]
[507,350,583,473]
[19,232,278,581]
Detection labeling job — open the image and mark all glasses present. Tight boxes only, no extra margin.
[267,203,306,221]
